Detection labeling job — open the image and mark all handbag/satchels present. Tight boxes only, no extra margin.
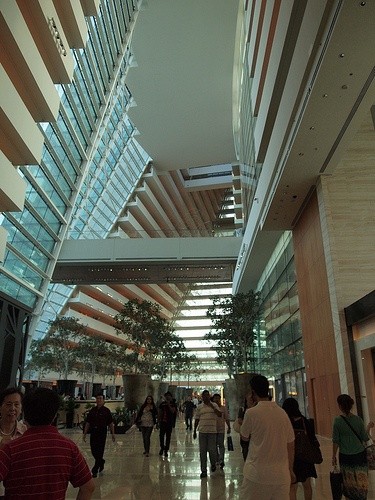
[329,464,343,500]
[233,407,245,433]
[365,444,375,470]
[227,435,234,451]
[300,438,323,464]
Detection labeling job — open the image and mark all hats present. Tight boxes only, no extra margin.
[164,391,173,397]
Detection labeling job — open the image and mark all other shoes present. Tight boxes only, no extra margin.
[220,463,224,469]
[200,472,207,477]
[164,450,168,457]
[211,464,216,472]
[92,473,97,478]
[146,452,148,456]
[216,459,219,465]
[159,448,164,455]
[186,425,189,430]
[190,426,192,431]
[99,460,105,472]
[144,451,146,454]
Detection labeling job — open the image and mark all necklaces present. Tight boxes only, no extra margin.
[0,426,16,436]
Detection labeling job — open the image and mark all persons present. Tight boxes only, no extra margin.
[156,390,232,468]
[282,398,318,500]
[332,394,374,500]
[155,391,177,456]
[239,375,295,500]
[137,395,157,457]
[193,389,222,477]
[83,394,116,478]
[0,387,95,500]
[0,387,28,496]
[237,391,257,462]
